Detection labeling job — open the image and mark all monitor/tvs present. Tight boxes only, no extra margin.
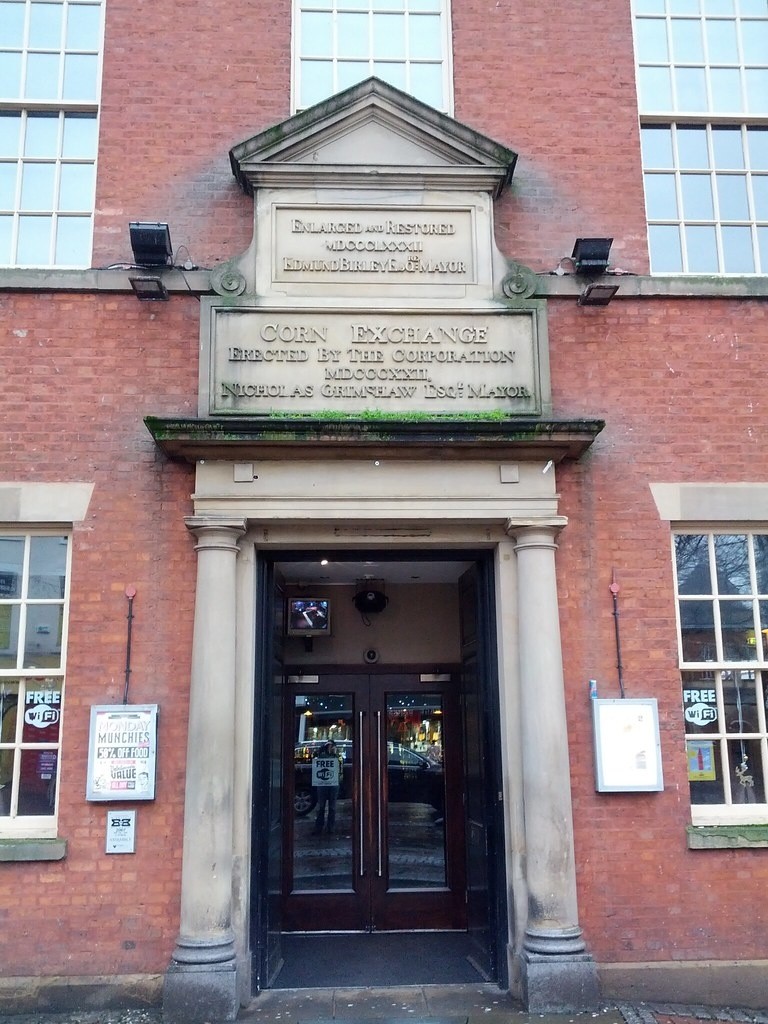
[286,598,331,636]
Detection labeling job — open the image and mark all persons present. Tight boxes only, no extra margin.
[0,694,23,785]
[310,740,344,837]
[392,749,402,763]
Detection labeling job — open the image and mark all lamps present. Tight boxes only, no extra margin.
[128,222,173,265]
[570,238,614,274]
[129,277,170,301]
[579,284,620,305]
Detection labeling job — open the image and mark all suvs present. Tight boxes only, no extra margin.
[292,740,445,820]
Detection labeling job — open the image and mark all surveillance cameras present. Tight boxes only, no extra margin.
[364,649,378,663]
